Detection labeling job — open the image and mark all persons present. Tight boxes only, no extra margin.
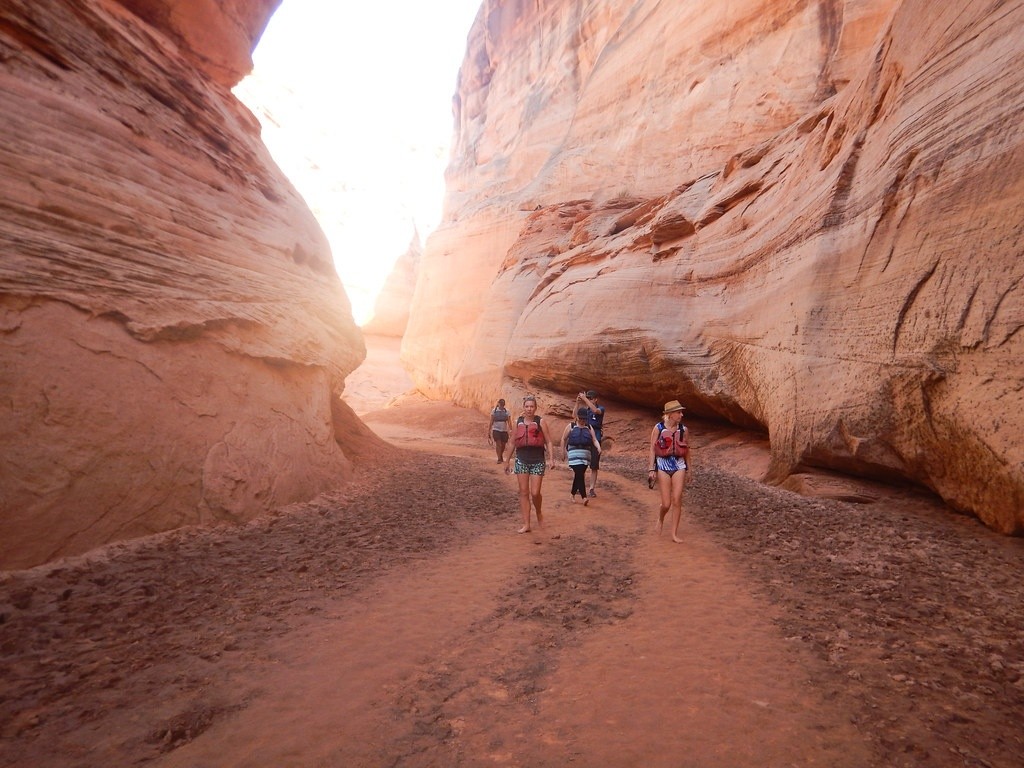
[572,391,604,497]
[504,398,555,534]
[648,401,692,543]
[488,399,513,464]
[561,407,602,506]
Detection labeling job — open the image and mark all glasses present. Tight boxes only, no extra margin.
[523,397,535,402]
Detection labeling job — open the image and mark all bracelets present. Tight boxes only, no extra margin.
[649,470,655,472]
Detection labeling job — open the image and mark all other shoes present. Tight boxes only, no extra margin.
[498,460,501,464]
[589,489,596,498]
[648,476,655,489]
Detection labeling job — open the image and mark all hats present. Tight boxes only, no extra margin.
[586,391,597,398]
[577,407,590,419]
[662,400,686,412]
[498,399,505,403]
